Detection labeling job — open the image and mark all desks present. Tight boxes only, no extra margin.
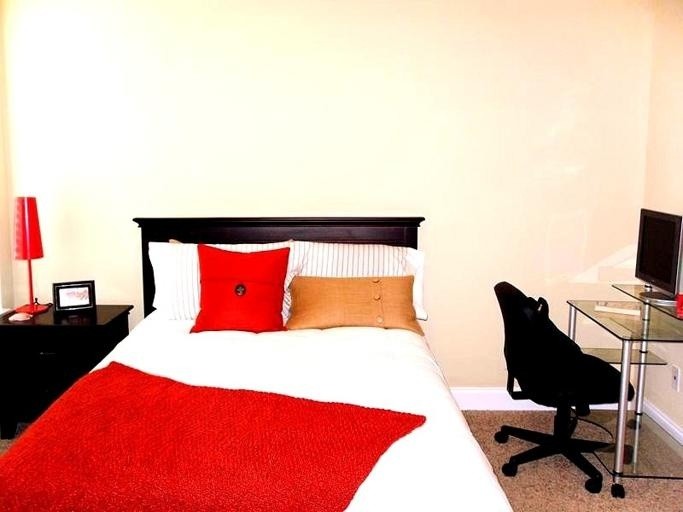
[567,284,682,484]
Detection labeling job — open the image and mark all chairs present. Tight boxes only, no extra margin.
[495,281,634,484]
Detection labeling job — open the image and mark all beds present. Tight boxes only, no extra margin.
[0,217,511,512]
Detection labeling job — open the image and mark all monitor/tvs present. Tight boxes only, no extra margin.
[635,209,682,303]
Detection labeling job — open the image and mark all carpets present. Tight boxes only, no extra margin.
[462,409,682,512]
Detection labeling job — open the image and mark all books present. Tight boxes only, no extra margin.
[596,301,643,316]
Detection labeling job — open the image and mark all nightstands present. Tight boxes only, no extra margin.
[2,306,130,438]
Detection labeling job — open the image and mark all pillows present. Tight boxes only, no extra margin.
[190,245,290,334]
[290,241,428,321]
[149,241,295,325]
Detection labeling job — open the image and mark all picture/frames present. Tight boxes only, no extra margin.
[52,279,96,313]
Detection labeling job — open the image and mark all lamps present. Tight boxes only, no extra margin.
[12,196,48,313]
